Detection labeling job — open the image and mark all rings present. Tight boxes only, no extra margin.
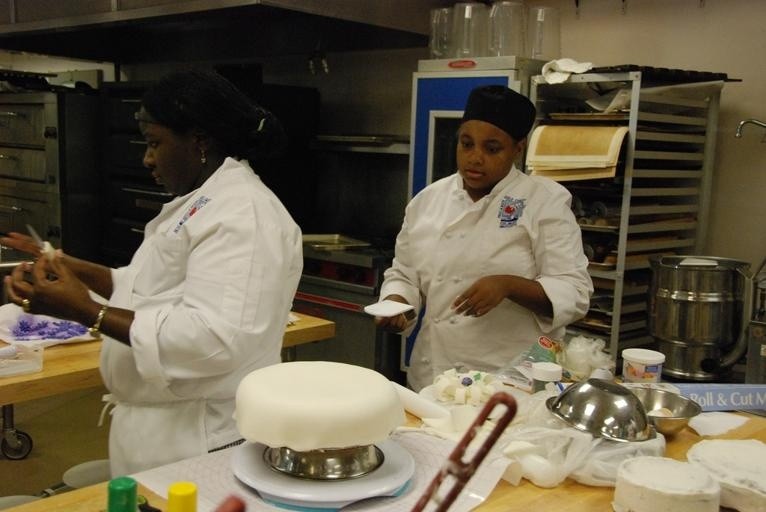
[22,298,29,309]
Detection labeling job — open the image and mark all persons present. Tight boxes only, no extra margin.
[373,84,595,394]
[1,68,306,478]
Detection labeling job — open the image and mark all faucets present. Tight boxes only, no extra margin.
[734,116,764,139]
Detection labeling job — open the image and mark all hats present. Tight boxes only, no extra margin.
[461,84,538,141]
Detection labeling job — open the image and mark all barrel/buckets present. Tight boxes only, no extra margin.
[645,254,759,383]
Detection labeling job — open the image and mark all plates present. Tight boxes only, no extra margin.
[231,437,414,504]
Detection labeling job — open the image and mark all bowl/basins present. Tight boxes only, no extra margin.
[544,377,657,442]
[624,384,703,437]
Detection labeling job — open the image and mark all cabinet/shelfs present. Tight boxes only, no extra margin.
[527,69,721,380]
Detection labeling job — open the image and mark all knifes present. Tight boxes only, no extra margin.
[24,221,58,262]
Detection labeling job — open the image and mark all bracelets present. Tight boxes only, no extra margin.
[88,305,107,338]
